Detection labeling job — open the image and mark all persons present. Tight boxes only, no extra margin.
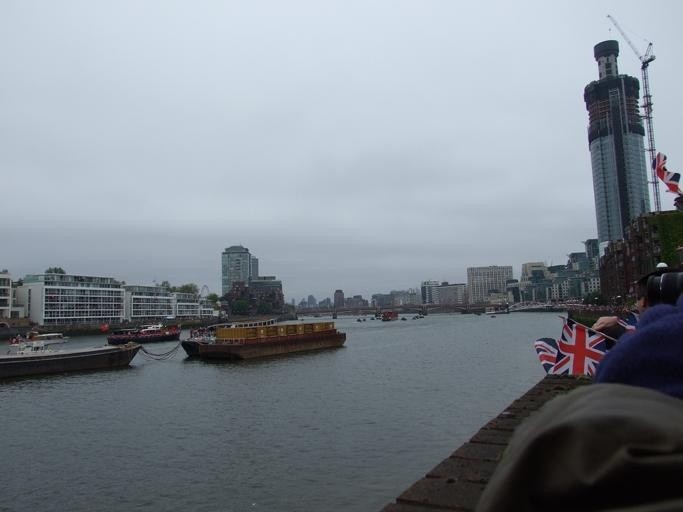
[7,334,24,354]
[591,270,683,398]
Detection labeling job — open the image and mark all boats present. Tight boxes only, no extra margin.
[382,311,398,321]
[8,331,71,348]
[0,341,141,379]
[138,324,166,332]
[107,325,181,345]
[181,321,347,361]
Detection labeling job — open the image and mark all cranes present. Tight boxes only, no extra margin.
[605,15,661,211]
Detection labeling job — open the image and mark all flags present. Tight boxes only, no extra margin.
[553,320,606,378]
[651,152,680,194]
[534,338,559,374]
